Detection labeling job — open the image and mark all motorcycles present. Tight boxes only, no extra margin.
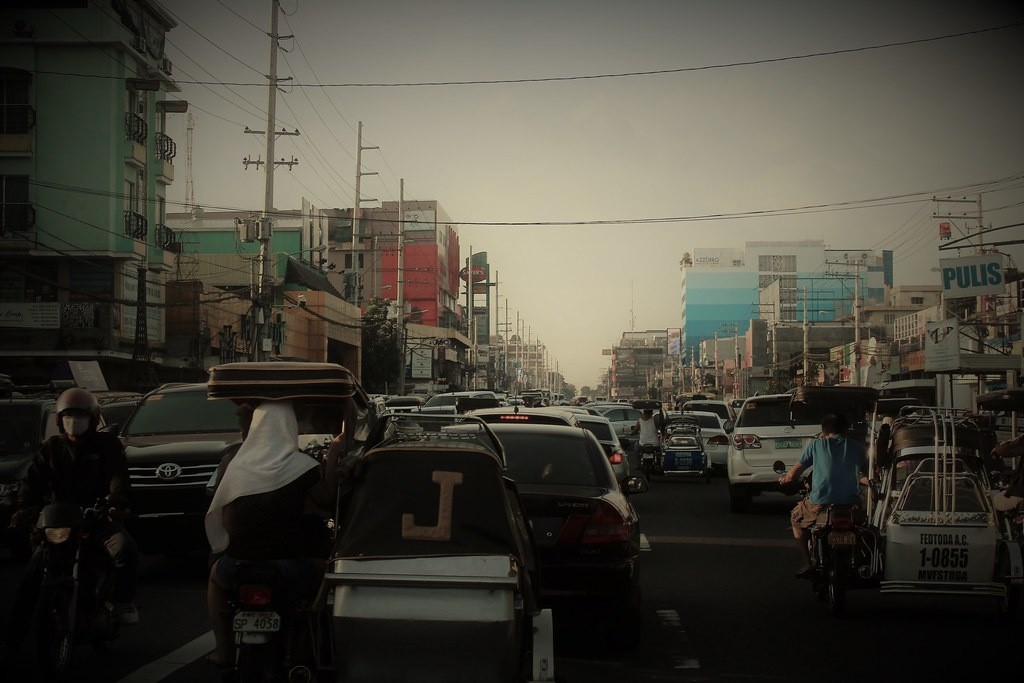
[0,493,121,683]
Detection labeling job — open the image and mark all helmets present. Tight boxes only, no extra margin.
[56,388,100,434]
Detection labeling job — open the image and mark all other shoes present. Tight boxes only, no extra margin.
[117,602,139,624]
[796,571,813,579]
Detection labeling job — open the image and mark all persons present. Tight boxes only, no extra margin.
[991,433,1024,490]
[877,417,893,470]
[777,417,869,575]
[205,401,348,669]
[23,389,138,623]
[633,410,660,467]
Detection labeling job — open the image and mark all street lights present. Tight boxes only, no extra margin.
[819,310,862,386]
[258,244,327,360]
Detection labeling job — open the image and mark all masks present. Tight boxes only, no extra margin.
[62,417,89,436]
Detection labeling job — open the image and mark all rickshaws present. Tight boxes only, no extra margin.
[206,361,555,683]
[632,398,712,484]
[975,388,1024,549]
[790,396,1024,620]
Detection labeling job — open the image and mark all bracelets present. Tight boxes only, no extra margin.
[330,445,337,449]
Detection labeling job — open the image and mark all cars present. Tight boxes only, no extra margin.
[0,361,1024,605]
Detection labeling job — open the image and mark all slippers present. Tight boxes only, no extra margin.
[205,653,230,671]
